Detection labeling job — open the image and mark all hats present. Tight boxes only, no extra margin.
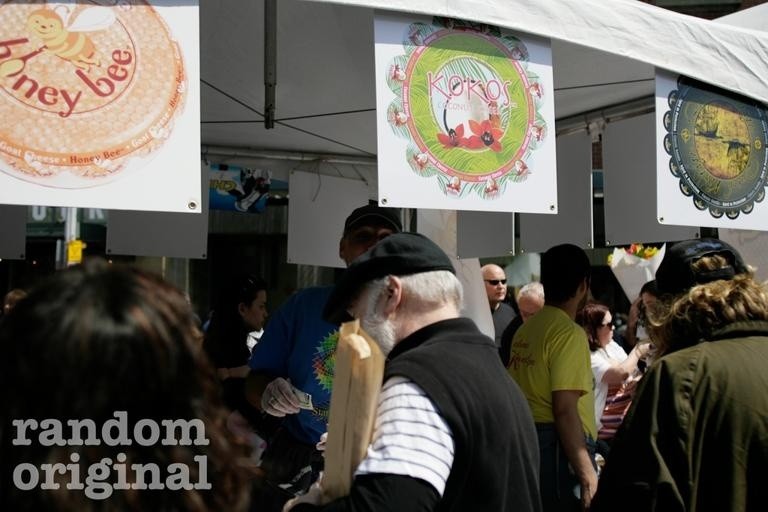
[321,232,456,326]
[655,238,749,300]
[345,204,402,236]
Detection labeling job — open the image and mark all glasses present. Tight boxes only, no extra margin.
[484,278,507,285]
[600,319,614,330]
